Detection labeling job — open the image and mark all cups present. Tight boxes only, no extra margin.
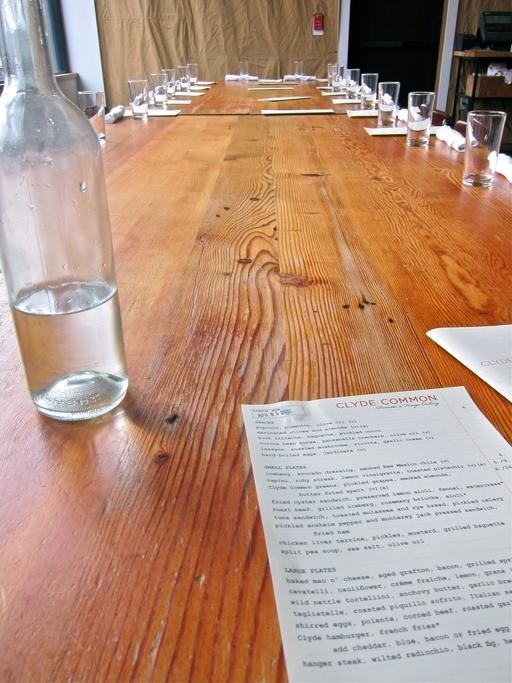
[151,62,197,106]
[406,90,438,148]
[295,62,304,81]
[327,63,379,110]
[239,60,249,80]
[461,108,507,186]
[77,88,107,143]
[377,82,403,128]
[129,79,150,121]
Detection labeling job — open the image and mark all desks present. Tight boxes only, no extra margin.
[123,79,379,113]
[0,114,512,683]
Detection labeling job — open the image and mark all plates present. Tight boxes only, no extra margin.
[486,63,512,85]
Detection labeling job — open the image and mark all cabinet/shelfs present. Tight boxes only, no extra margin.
[445,45,512,151]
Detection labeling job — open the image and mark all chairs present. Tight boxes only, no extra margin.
[81,105,110,120]
[421,104,450,125]
[453,119,487,143]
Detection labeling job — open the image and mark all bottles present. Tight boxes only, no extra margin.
[0,1,130,422]
